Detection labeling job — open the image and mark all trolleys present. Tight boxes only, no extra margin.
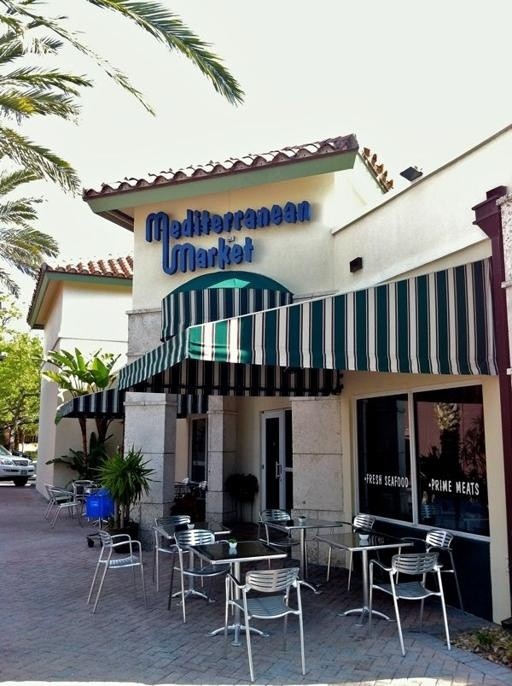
[85,491,115,548]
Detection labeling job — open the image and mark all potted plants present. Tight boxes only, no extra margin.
[87,442,160,553]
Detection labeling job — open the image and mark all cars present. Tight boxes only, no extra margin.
[0,444,36,487]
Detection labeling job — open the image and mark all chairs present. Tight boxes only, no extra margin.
[257,508,301,570]
[154,515,193,593]
[367,552,453,658]
[43,476,96,530]
[395,528,464,624]
[173,528,232,624]
[222,566,308,682]
[326,513,376,591]
[85,529,148,615]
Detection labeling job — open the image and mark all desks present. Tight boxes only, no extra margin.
[188,540,289,647]
[261,517,343,596]
[313,532,416,627]
[152,521,230,606]
